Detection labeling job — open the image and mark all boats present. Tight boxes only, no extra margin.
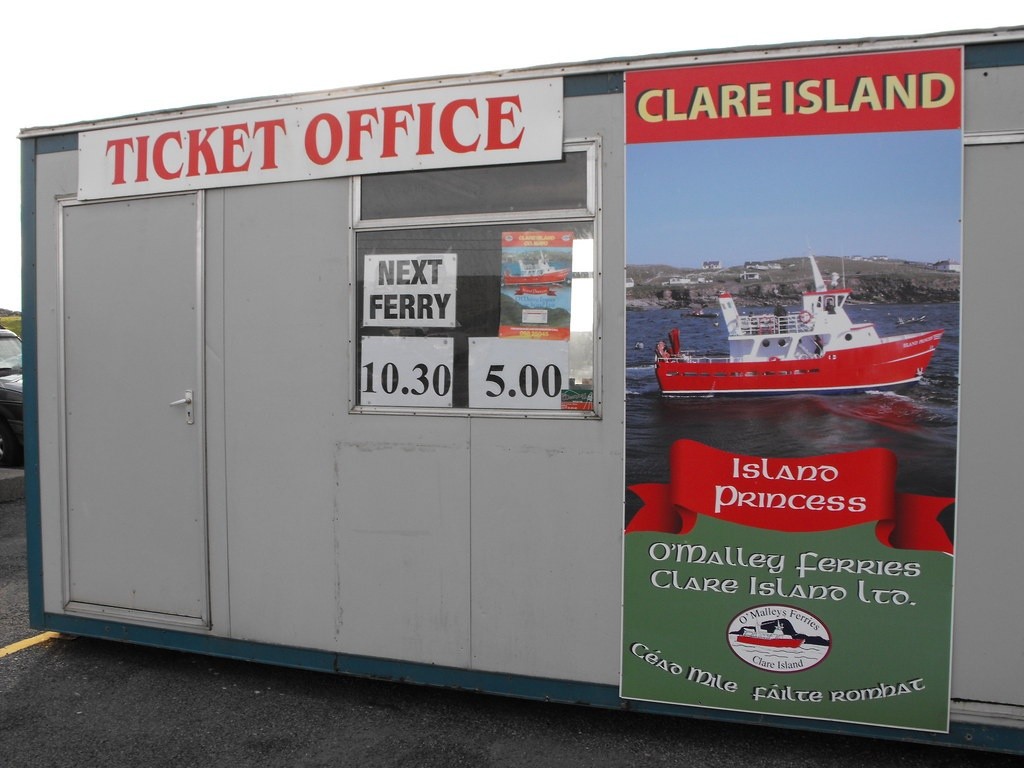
[653,256,945,399]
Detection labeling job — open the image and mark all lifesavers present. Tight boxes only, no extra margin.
[799,310,814,324]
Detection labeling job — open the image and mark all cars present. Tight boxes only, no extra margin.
[0,327,26,465]
